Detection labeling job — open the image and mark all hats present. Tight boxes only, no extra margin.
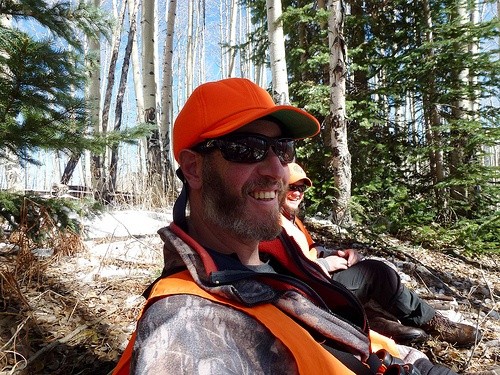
[283,161,312,190]
[172,77,320,161]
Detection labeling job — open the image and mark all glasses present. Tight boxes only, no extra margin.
[202,130,296,164]
[289,183,306,193]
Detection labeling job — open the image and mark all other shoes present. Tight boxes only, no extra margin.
[372,317,426,349]
[425,312,483,348]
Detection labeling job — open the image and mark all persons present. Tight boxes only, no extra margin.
[111,78,458,375]
[275,163,483,348]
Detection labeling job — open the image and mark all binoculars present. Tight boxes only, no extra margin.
[374,350,421,375]
